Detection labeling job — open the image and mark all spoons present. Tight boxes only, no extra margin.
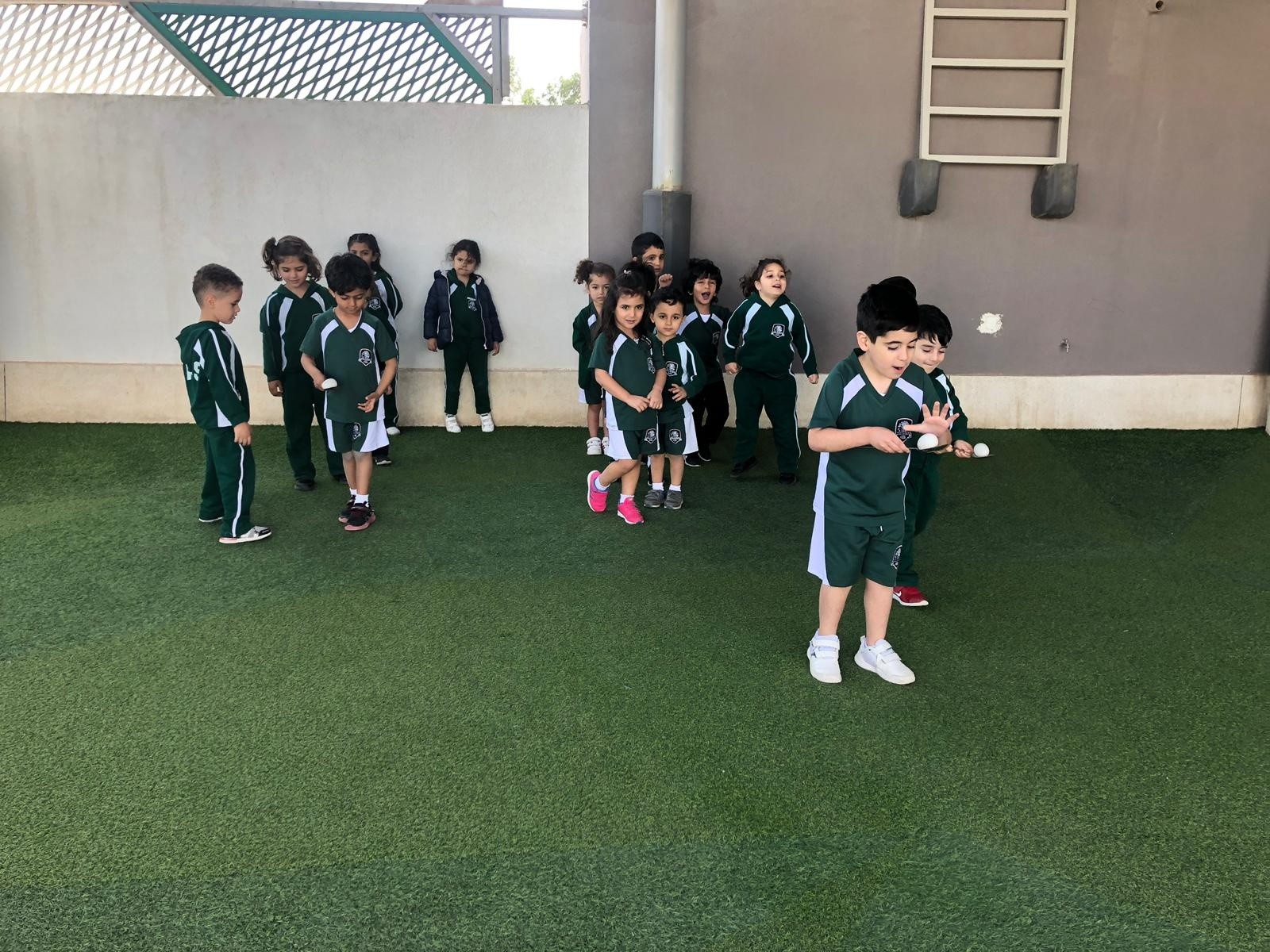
[858,443,948,452]
[972,453,997,459]
[324,386,337,391]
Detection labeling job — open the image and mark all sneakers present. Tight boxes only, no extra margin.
[344,501,376,530]
[664,489,684,508]
[338,494,356,523]
[854,636,915,684]
[617,497,644,524]
[807,628,842,684]
[893,586,929,606]
[199,516,223,522]
[644,489,664,507]
[587,470,609,511]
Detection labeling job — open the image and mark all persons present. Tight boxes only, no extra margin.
[808,282,960,686]
[259,236,348,490]
[723,259,819,485]
[878,276,974,606]
[424,240,505,433]
[345,234,403,464]
[646,287,707,509]
[175,263,273,543]
[572,231,731,481]
[300,252,400,531]
[588,275,666,524]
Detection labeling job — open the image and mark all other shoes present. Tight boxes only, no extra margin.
[685,455,702,467]
[375,456,392,465]
[294,478,317,491]
[333,474,347,484]
[387,427,400,435]
[729,455,760,478]
[445,414,461,433]
[777,473,797,485]
[218,526,272,543]
[602,437,610,455]
[586,437,602,455]
[647,464,665,486]
[610,457,621,482]
[479,412,494,432]
[697,425,712,461]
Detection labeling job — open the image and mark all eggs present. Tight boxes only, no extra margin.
[917,433,939,449]
[322,378,337,389]
[974,443,989,457]
